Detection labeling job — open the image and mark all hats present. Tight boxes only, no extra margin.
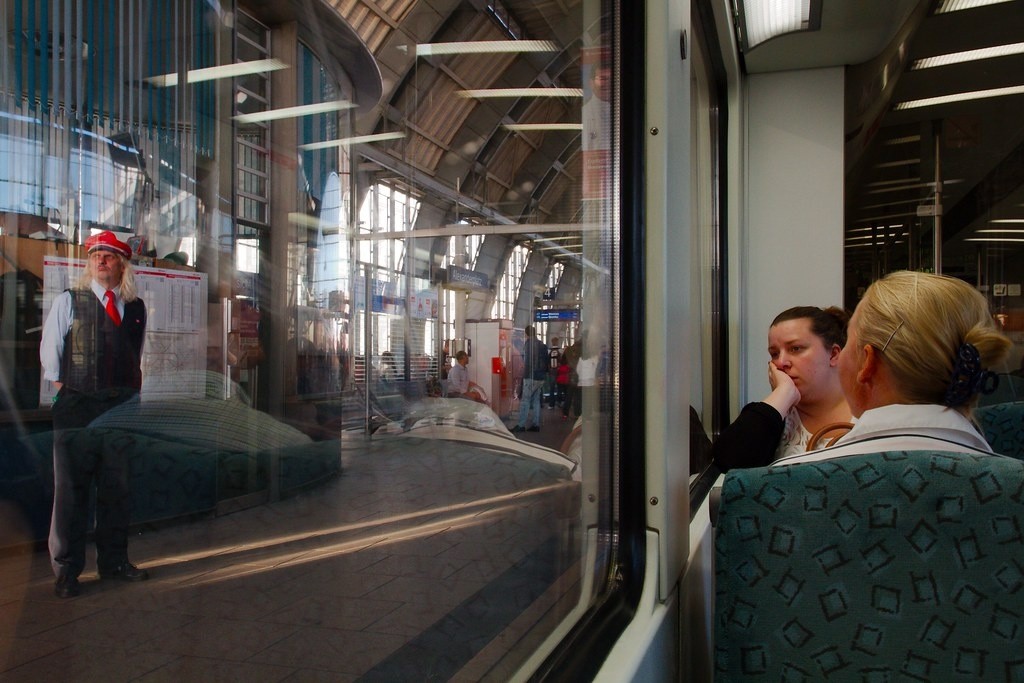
[84,230,132,262]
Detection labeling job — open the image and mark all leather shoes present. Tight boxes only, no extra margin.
[54,576,84,598]
[98,563,150,584]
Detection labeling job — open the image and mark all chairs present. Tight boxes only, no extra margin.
[12,420,573,532]
[714,448,1024,683]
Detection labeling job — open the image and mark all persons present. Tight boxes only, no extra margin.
[714,271,1016,475]
[690,405,713,474]
[373,306,582,482]
[508,326,550,434]
[555,331,612,420]
[353,351,439,384]
[86,264,314,453]
[40,231,148,598]
[446,351,489,405]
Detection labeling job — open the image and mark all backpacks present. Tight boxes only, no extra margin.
[548,349,562,372]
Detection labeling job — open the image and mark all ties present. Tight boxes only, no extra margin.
[104,290,122,325]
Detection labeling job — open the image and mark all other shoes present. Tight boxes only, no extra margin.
[547,405,553,409]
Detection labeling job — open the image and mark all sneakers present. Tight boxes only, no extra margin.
[509,425,525,432]
[562,415,566,421]
[528,425,540,432]
